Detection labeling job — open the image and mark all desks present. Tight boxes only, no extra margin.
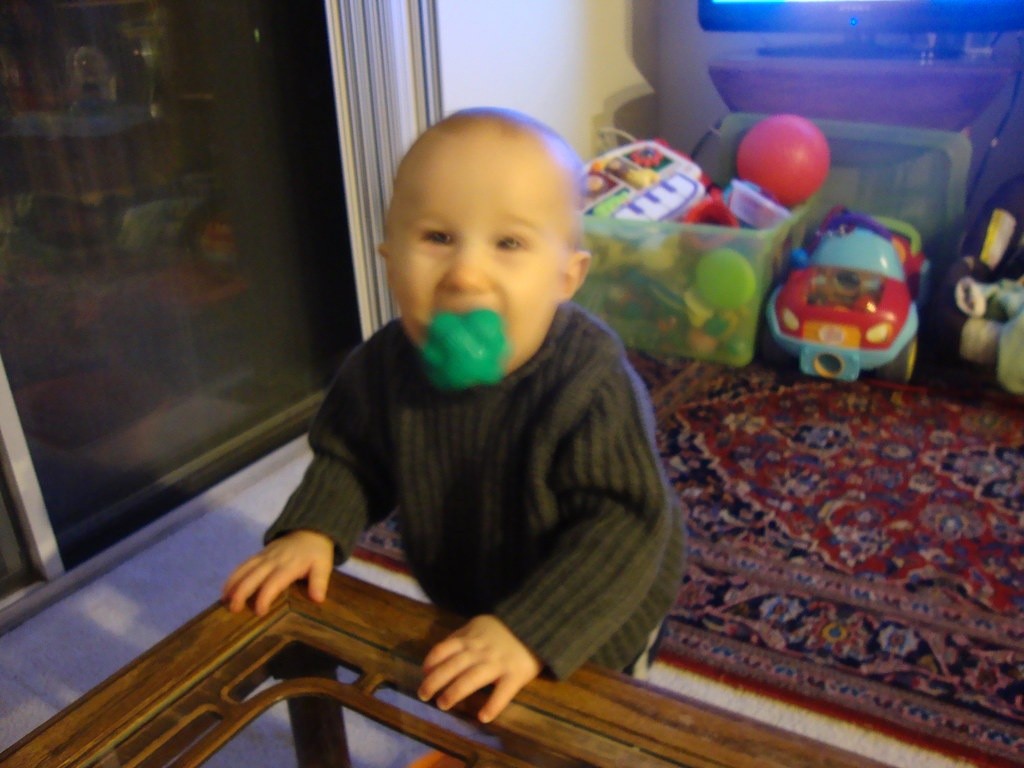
[2,567,904,768]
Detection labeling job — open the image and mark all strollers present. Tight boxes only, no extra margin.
[3,27,244,321]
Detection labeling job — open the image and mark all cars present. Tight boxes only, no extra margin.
[762,203,935,385]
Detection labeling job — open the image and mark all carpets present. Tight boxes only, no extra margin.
[343,350,1024,767]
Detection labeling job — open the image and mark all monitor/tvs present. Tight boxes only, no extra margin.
[697,0,1024,61]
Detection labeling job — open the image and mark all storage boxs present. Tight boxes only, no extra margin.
[581,190,806,370]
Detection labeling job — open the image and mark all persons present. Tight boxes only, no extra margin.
[219,107,686,724]
